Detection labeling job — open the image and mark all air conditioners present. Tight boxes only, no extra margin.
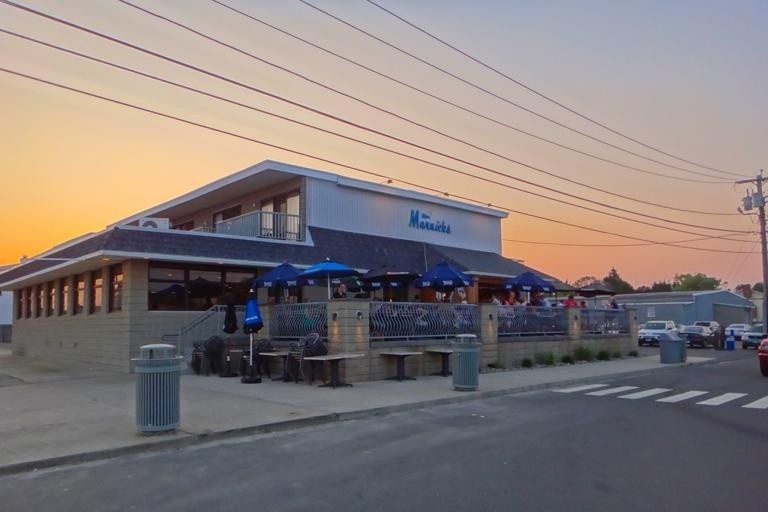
[139,218,169,229]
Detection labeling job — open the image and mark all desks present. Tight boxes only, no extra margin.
[303,354,365,389]
[426,350,453,377]
[259,351,303,383]
[380,352,423,382]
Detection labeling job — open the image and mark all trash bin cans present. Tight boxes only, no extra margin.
[452,334,482,391]
[131,344,184,433]
[656,331,687,363]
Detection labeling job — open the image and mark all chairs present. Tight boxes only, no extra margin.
[370,305,627,342]
[240,339,272,383]
[283,332,328,386]
[192,336,225,376]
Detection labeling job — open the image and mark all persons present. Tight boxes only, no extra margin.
[604,294,618,331]
[265,293,589,343]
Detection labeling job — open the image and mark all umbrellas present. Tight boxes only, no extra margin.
[243,287,263,377]
[346,278,402,297]
[413,259,475,302]
[362,263,420,282]
[295,256,361,299]
[221,294,240,372]
[251,259,320,288]
[504,269,555,303]
[316,276,343,296]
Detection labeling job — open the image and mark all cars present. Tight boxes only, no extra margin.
[638,321,768,377]
